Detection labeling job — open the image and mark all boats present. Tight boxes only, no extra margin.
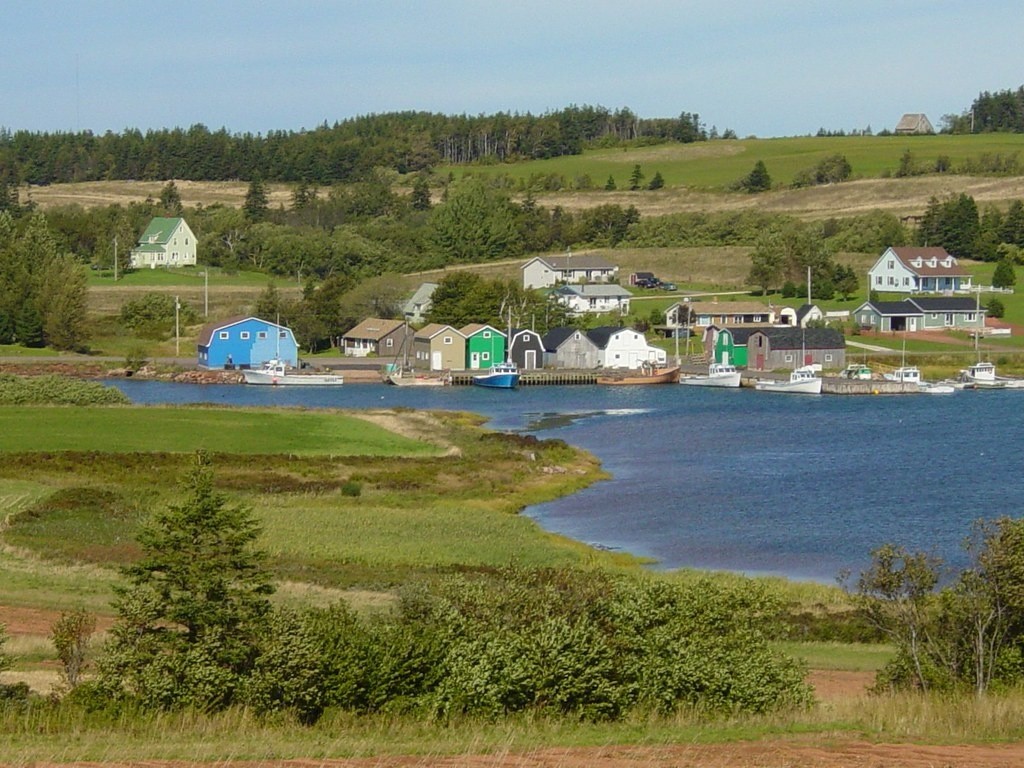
[679,363,741,388]
[840,361,1009,393]
[596,360,682,385]
[472,363,522,390]
[755,369,823,394]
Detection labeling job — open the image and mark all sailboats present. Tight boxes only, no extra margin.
[388,319,452,387]
[241,312,345,385]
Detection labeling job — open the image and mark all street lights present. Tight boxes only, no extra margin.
[566,246,574,286]
[684,297,690,357]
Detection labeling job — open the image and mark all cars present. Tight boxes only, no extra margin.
[637,276,678,291]
[90,262,114,270]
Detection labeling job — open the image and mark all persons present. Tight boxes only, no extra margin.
[228,354,232,363]
